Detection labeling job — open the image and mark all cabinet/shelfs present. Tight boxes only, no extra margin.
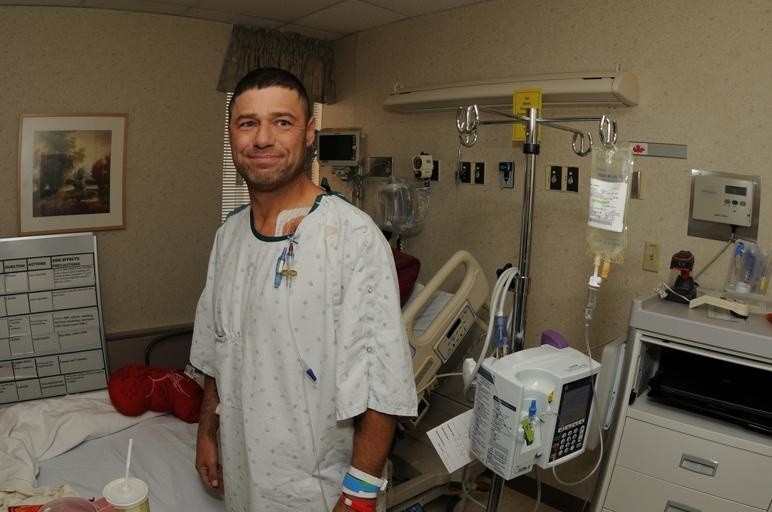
[591,293,772,511]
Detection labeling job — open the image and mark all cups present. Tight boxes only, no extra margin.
[102,476,150,512]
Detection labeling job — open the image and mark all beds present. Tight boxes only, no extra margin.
[0,250,489,511]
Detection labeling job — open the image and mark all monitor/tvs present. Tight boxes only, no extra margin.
[316,127,366,167]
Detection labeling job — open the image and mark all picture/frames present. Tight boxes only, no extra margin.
[15,111,129,236]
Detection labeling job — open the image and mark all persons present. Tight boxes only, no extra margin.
[187,67,420,512]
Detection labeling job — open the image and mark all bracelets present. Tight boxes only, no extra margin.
[340,464,389,512]
